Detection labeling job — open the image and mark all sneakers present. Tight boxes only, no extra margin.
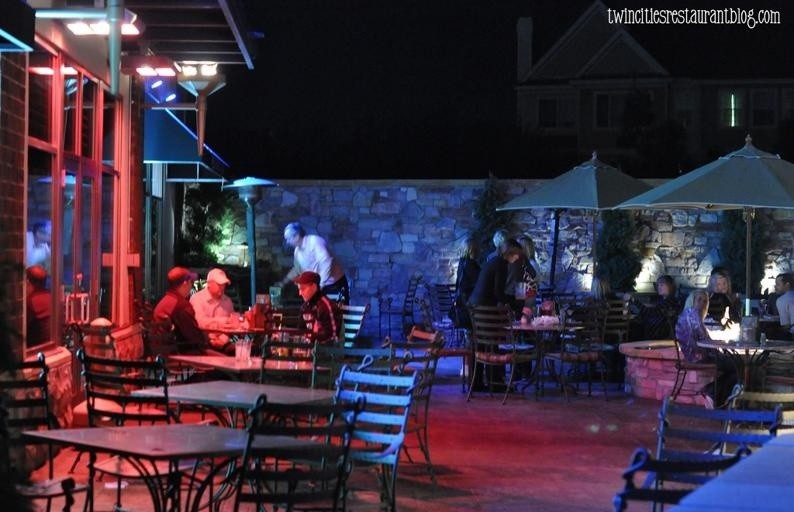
[468,384,487,392]
[487,384,515,393]
[704,385,714,409]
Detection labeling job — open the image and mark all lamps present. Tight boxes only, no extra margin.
[174,59,221,81]
[223,176,280,308]
[63,8,143,38]
[125,55,175,78]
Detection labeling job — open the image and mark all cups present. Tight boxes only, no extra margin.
[236,339,252,366]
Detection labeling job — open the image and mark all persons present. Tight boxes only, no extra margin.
[622,276,682,339]
[449,237,487,329]
[188,269,245,357]
[511,235,540,379]
[275,223,348,314]
[578,275,620,335]
[293,272,338,361]
[764,275,794,338]
[468,241,525,393]
[27,222,50,271]
[677,288,743,408]
[706,267,740,325]
[152,266,204,355]
[487,229,511,260]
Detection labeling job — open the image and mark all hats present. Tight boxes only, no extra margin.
[293,272,320,284]
[207,268,231,285]
[168,267,198,285]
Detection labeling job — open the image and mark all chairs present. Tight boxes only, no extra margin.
[699,384,794,476]
[305,344,381,443]
[335,298,370,339]
[591,304,628,391]
[668,335,719,408]
[467,305,537,405]
[292,365,415,512]
[71,321,127,398]
[259,340,334,438]
[581,310,606,395]
[0,352,92,512]
[652,398,780,512]
[77,351,215,512]
[378,274,425,340]
[237,395,365,512]
[426,284,462,348]
[299,354,417,510]
[547,306,583,399]
[612,445,752,512]
[383,344,438,499]
[133,299,174,351]
